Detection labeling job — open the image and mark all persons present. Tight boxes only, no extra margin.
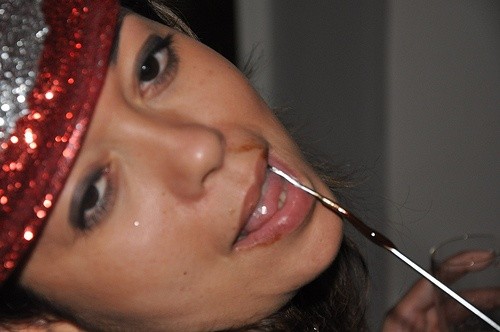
[0,0,500,332]
